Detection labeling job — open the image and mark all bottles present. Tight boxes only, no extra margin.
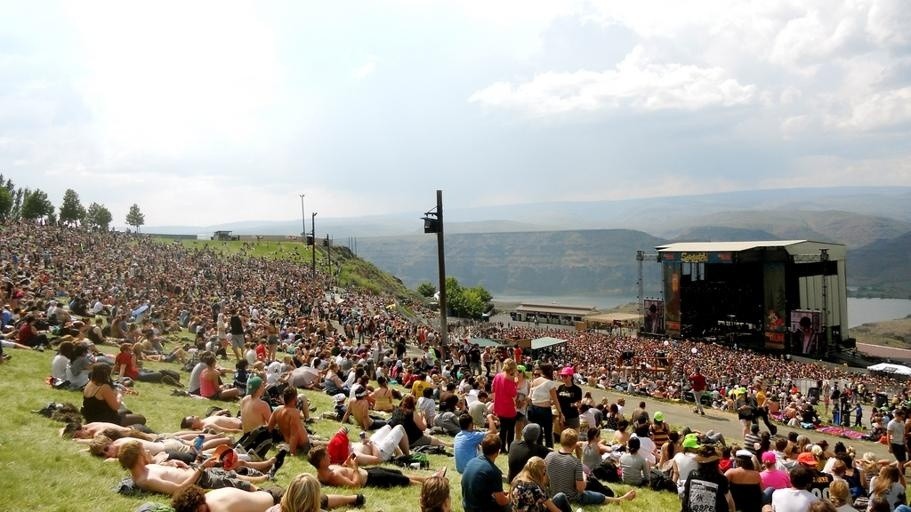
[194,434,205,450]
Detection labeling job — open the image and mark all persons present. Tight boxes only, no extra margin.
[2,215,493,414]
[62,362,635,510]
[445,317,911,511]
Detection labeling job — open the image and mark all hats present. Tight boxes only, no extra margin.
[245,377,261,394]
[516,364,526,373]
[692,446,721,463]
[522,424,540,443]
[682,433,700,450]
[654,410,663,422]
[559,366,573,376]
[736,449,753,458]
[211,445,238,470]
[738,386,745,394]
[798,452,819,466]
[761,452,776,464]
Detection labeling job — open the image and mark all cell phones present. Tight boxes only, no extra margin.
[349,453,357,461]
